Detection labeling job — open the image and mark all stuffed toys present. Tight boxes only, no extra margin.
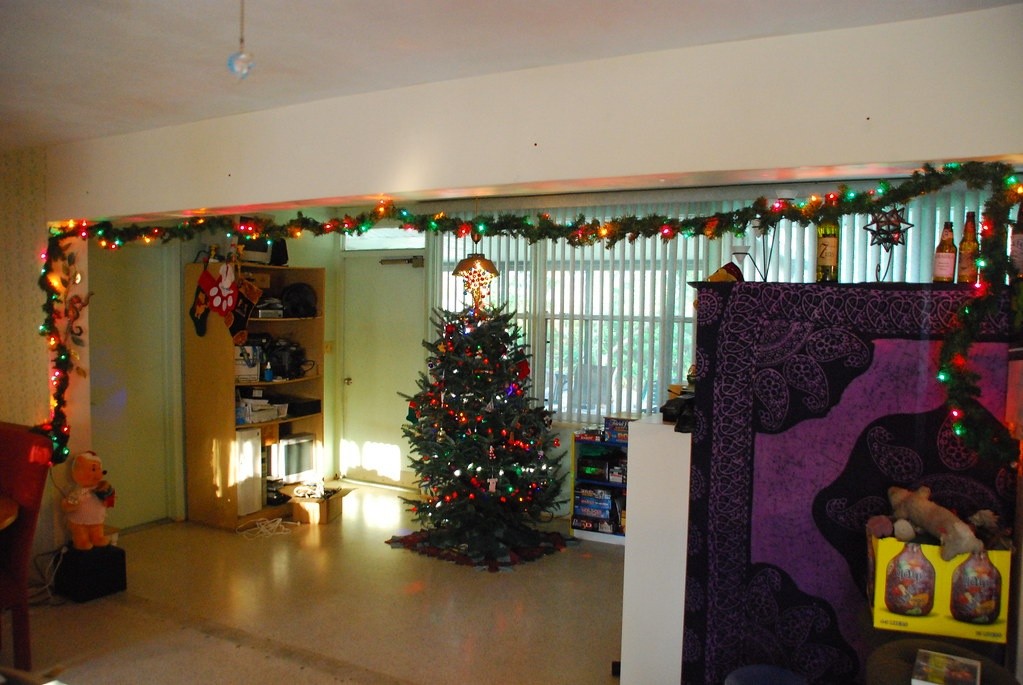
[888,487,983,561]
[62,451,115,550]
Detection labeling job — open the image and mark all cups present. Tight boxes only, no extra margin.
[274,404,288,417]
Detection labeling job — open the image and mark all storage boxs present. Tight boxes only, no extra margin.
[54,544,127,605]
[233,347,261,382]
[246,386,266,399]
[572,418,631,535]
[280,484,358,524]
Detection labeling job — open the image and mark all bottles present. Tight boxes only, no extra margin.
[1009,202,1023,284]
[235,387,244,425]
[957,212,979,283]
[817,216,840,283]
[933,222,956,282]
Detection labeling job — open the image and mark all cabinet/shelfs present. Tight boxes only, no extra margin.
[568,411,652,544]
[620,414,692,685]
[183,261,327,532]
